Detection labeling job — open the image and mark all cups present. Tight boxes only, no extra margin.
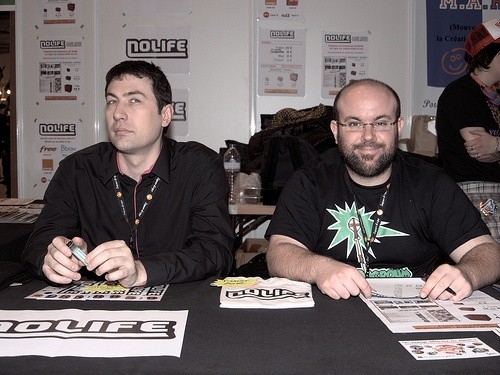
[244,186,262,204]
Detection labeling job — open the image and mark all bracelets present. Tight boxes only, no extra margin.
[492,135,499,157]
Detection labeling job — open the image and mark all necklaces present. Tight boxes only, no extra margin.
[471,72,500,105]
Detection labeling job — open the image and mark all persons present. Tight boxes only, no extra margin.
[436,19,500,182]
[264,78,500,302]
[24,61,237,286]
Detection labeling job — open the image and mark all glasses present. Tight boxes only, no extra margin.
[337,119,398,132]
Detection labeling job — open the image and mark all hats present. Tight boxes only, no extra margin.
[464,18,500,67]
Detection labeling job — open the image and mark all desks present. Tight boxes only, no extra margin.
[0,198,500,375]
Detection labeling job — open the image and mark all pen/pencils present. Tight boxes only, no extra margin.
[420,271,457,297]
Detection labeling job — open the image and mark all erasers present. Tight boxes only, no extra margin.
[65,239,91,266]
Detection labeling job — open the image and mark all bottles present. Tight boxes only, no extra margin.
[223,143,241,205]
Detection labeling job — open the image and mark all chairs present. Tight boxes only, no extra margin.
[225,107,318,247]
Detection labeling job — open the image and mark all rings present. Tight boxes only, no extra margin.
[471,145,473,150]
[476,152,480,158]
